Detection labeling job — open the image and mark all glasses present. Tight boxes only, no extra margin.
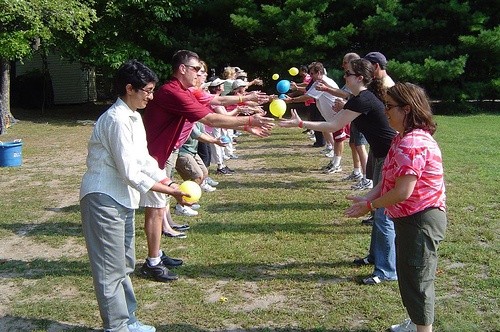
[132,84,155,96]
[310,72,318,75]
[385,104,405,111]
[185,65,201,71]
[345,70,361,77]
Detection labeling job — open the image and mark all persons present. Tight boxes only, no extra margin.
[163,62,278,239]
[278,58,399,285]
[282,51,395,189]
[344,83,448,332]
[140,50,275,282]
[80,61,194,332]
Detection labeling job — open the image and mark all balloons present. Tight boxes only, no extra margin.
[276,80,290,93]
[288,67,299,76]
[180,181,202,202]
[279,94,286,100]
[272,74,279,80]
[269,99,286,118]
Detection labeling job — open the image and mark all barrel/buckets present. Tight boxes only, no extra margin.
[0,140,23,167]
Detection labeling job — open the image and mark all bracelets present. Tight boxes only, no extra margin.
[370,203,377,210]
[249,117,252,126]
[239,96,242,103]
[168,182,174,187]
[325,88,328,91]
[299,121,303,128]
[244,125,247,130]
[368,201,371,209]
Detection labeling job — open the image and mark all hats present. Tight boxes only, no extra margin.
[232,67,247,90]
[209,77,227,86]
[364,51,388,66]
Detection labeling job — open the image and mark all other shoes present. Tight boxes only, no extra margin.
[362,274,385,285]
[353,257,374,265]
[162,231,187,238]
[170,224,190,231]
[362,217,376,226]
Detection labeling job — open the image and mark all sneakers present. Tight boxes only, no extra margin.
[140,259,179,282]
[390,318,417,332]
[190,204,200,210]
[200,131,242,192]
[160,251,183,269]
[176,203,198,216]
[302,128,343,174]
[128,321,156,332]
[342,171,364,182]
[351,178,374,191]
